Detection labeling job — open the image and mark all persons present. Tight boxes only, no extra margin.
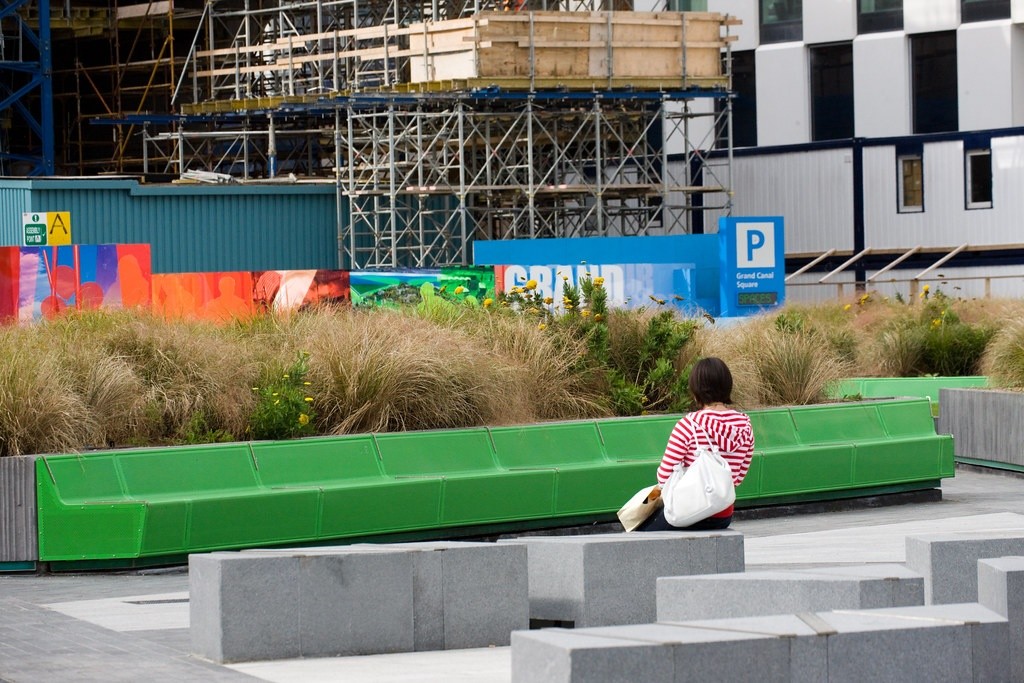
[636,357,754,531]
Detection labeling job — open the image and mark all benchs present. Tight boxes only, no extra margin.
[977,556,1024,683]
[188,541,529,665]
[657,565,924,623]
[511,602,1010,683]
[497,530,745,628]
[821,374,992,435]
[36,397,954,578]
[906,532,1024,606]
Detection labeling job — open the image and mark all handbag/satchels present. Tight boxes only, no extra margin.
[616,483,661,532]
[660,415,736,527]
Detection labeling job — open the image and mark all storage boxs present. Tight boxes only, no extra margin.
[409,12,721,84]
